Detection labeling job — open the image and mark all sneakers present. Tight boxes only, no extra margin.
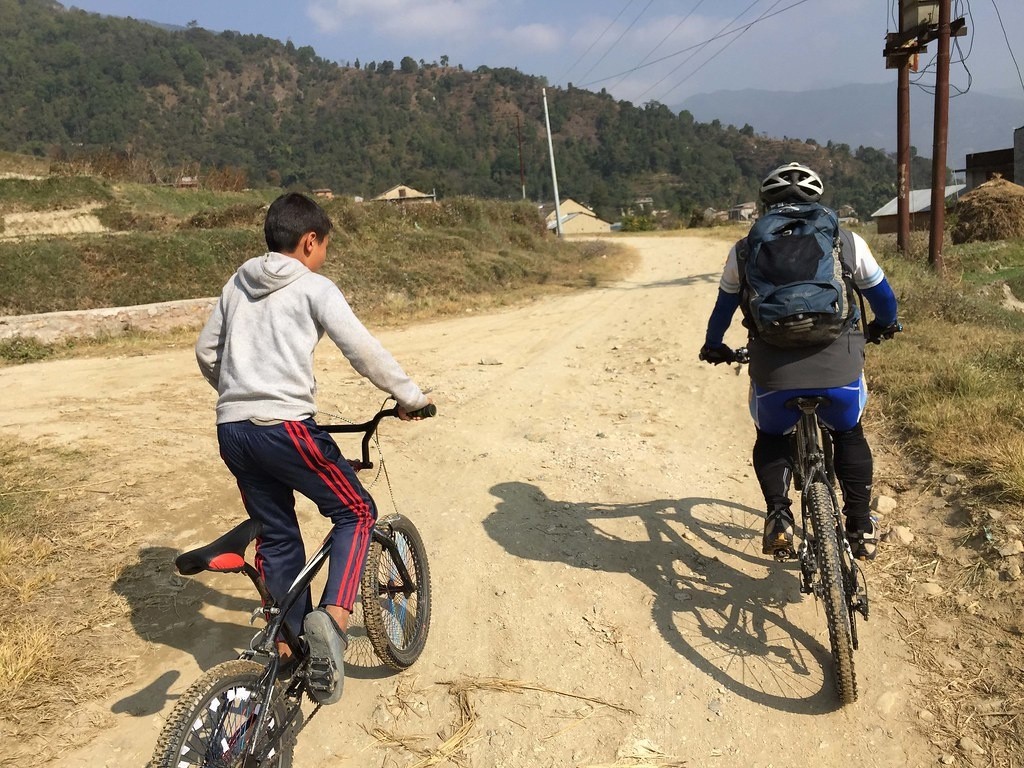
[846,517,879,562]
[762,508,794,558]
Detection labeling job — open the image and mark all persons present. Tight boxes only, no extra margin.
[701,161,899,560]
[196,192,433,705]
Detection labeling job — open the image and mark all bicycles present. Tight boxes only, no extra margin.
[150,393,437,768]
[698,323,903,706]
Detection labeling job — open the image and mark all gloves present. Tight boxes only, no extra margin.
[866,320,897,345]
[698,340,733,367]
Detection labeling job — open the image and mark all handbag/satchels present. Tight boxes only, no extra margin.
[742,201,871,347]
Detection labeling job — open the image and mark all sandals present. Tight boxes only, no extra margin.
[305,608,349,706]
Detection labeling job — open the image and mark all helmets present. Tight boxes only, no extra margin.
[759,160,825,205]
